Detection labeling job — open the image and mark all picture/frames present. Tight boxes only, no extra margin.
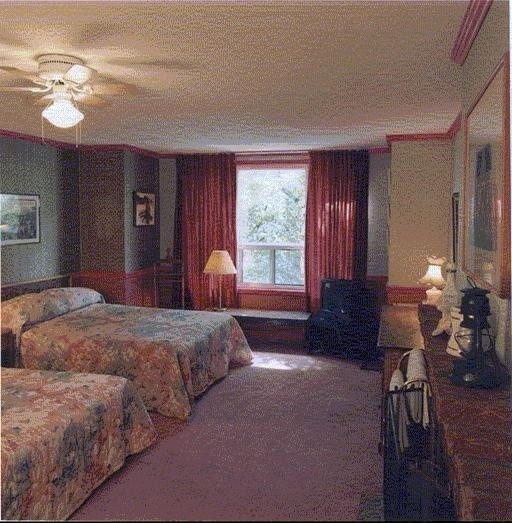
[133,189,158,227]
[461,49,511,297]
[0,194,41,245]
[451,191,461,263]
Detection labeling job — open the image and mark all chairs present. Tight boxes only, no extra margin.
[306,273,384,371]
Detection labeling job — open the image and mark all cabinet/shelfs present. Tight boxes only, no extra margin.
[206,307,313,356]
[139,254,189,309]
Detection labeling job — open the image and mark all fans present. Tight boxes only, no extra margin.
[0,54,138,107]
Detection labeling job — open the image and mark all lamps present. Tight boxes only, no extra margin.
[449,275,511,389]
[202,250,238,311]
[417,254,447,305]
[37,80,86,130]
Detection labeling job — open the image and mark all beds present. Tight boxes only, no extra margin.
[0,365,160,521]
[1,286,252,420]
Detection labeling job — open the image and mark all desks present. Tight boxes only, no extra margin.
[419,304,511,522]
[375,302,430,453]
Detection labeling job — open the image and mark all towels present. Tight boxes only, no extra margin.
[386,347,436,451]
